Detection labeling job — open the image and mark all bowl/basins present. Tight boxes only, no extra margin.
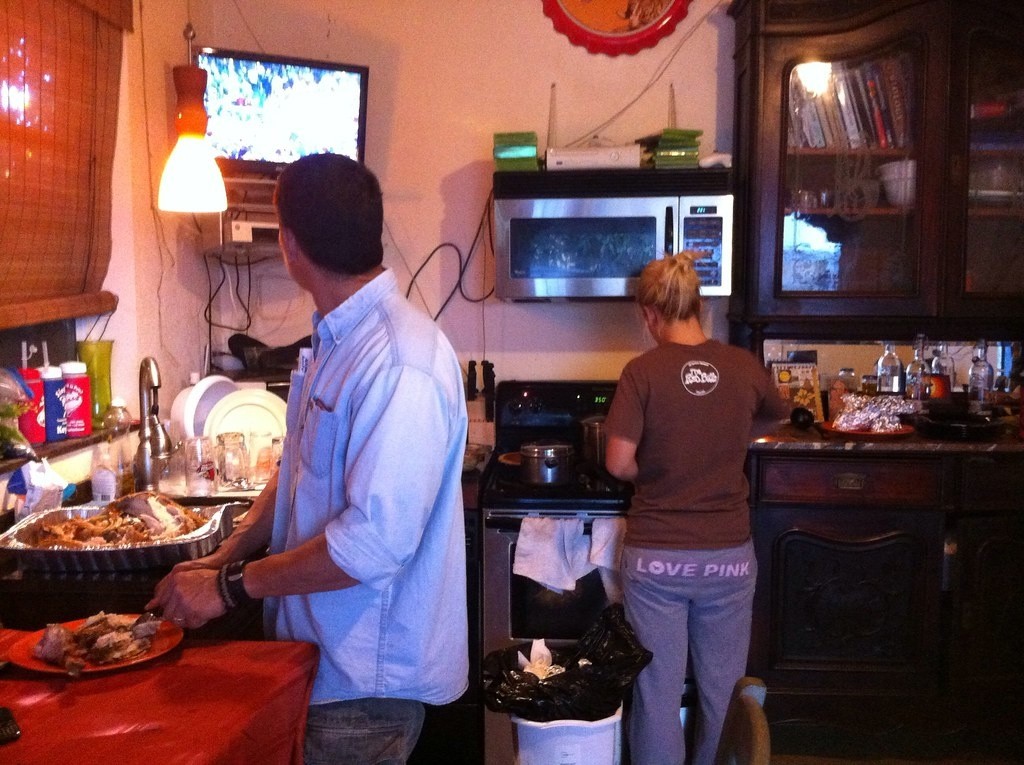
[463,443,493,471]
[169,375,238,438]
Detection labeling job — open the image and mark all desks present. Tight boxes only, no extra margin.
[0,627,321,765]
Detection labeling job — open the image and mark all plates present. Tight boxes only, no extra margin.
[203,387,288,467]
[498,451,521,466]
[911,413,1004,440]
[820,420,915,440]
[7,613,184,674]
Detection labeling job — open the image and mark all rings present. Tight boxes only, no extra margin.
[172,611,185,621]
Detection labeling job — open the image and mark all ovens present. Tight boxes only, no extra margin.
[482,507,697,765]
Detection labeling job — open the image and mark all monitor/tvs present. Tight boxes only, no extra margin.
[193,46,369,177]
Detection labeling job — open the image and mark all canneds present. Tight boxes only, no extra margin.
[861,375,877,396]
[837,368,856,396]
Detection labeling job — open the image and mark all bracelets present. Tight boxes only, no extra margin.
[213,559,255,613]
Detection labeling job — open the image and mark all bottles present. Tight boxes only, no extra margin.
[840,334,995,404]
[16,360,91,448]
[102,399,136,498]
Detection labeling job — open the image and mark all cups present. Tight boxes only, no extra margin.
[185,433,285,495]
[76,339,115,430]
[878,159,915,206]
[792,187,839,208]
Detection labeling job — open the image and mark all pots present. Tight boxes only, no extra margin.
[519,438,574,486]
[580,415,607,468]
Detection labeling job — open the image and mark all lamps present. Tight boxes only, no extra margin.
[156,0,229,214]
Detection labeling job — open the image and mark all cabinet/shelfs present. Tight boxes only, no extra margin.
[746,423,1024,760]
[725,0,1023,343]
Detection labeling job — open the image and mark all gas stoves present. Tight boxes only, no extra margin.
[481,380,637,509]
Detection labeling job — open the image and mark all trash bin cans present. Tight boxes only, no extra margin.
[483,643,623,765]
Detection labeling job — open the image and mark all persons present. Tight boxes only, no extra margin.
[144,154,471,765]
[605,250,784,765]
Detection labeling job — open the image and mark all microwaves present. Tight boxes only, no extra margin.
[492,168,734,302]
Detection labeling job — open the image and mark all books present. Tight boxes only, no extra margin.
[787,55,1024,217]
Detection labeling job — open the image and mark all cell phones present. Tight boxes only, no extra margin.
[0,707,22,743]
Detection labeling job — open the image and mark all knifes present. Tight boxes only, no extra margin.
[467,360,496,422]
[129,606,163,629]
